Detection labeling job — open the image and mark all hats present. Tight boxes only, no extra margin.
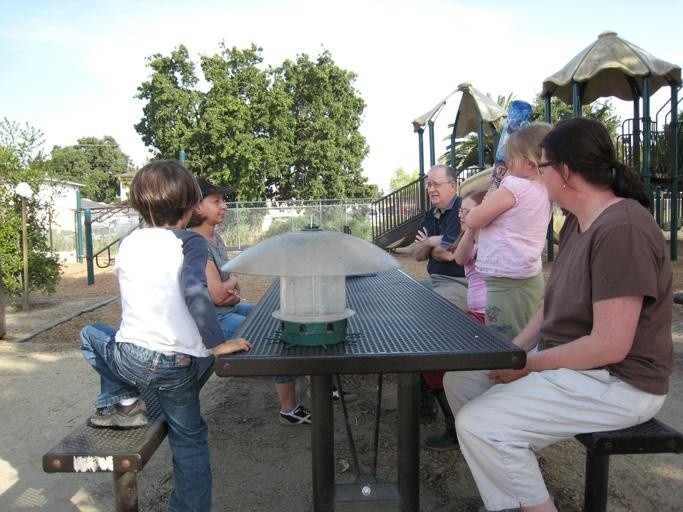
[195,176,235,197]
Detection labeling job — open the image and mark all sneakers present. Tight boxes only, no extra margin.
[307,385,359,404]
[90,398,148,428]
[279,404,312,427]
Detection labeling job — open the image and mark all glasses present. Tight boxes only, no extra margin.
[537,162,553,174]
[458,209,469,215]
[427,182,451,188]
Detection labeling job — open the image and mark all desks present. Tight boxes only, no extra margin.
[228,267,527,511]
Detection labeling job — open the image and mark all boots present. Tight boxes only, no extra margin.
[419,373,439,423]
[423,388,460,451]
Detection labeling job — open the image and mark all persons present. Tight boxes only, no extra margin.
[442,118,675,512]
[464,122,553,346]
[417,188,489,452]
[186,177,355,427]
[79,161,254,511]
[411,164,470,316]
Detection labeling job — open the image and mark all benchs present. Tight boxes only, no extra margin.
[42,363,214,510]
[572,417,682,510]
